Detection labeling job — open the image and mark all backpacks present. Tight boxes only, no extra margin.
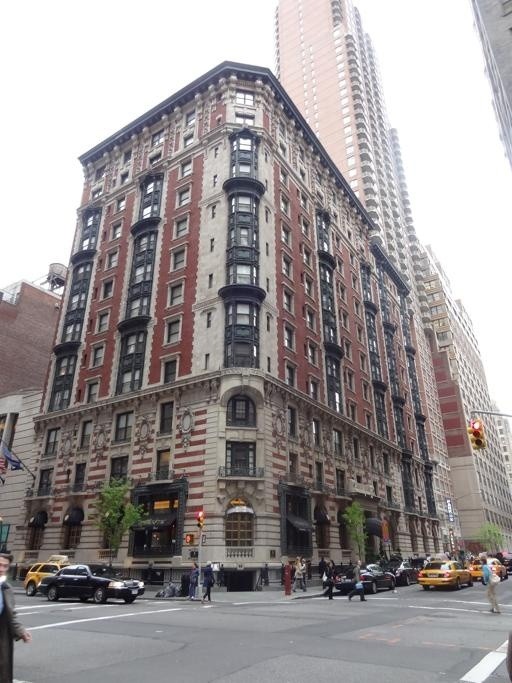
[348,565,357,578]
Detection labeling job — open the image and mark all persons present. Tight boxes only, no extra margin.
[480,553,501,615]
[186,561,200,600]
[265,556,367,601]
[0,554,32,681]
[203,562,215,602]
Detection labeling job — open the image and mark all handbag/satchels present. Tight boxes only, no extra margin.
[488,574,501,586]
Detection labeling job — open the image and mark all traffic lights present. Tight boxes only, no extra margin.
[467,419,485,450]
[186,534,193,543]
[198,512,205,527]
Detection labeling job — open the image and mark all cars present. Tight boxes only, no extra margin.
[336,564,396,594]
[24,562,144,603]
[389,552,511,591]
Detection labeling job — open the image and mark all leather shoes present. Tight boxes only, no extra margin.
[349,592,351,599]
[361,598,367,600]
